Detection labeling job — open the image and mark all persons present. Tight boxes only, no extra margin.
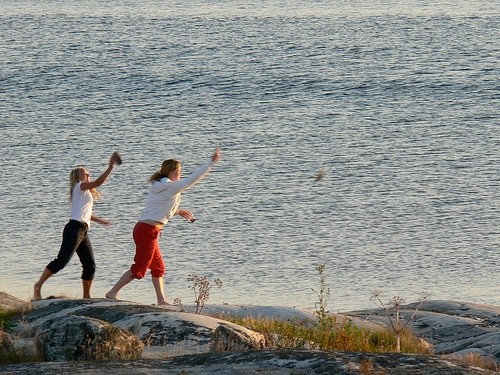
[32,156,117,301]
[105,147,221,306]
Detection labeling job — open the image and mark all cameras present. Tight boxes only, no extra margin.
[186,211,197,223]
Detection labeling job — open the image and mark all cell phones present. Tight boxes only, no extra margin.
[113,151,122,165]
